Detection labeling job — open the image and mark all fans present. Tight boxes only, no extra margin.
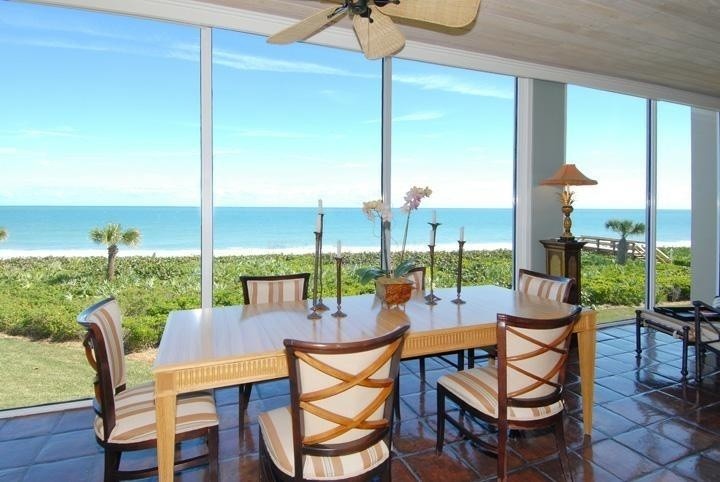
[266,0,482,59]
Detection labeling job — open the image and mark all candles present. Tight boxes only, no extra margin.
[429,230,434,245]
[318,199,322,213]
[432,210,436,224]
[459,226,464,241]
[317,214,321,232]
[337,240,341,258]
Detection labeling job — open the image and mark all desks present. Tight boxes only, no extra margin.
[151,284,597,482]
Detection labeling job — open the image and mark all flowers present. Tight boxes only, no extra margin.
[355,186,432,286]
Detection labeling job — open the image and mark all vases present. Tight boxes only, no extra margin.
[373,276,414,310]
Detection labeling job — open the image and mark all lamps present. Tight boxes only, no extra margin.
[539,164,598,242]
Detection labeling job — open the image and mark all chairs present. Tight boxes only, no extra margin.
[259,324,411,481]
[468,268,577,372]
[239,273,311,438]
[436,308,581,482]
[76,297,220,482]
[375,267,464,421]
